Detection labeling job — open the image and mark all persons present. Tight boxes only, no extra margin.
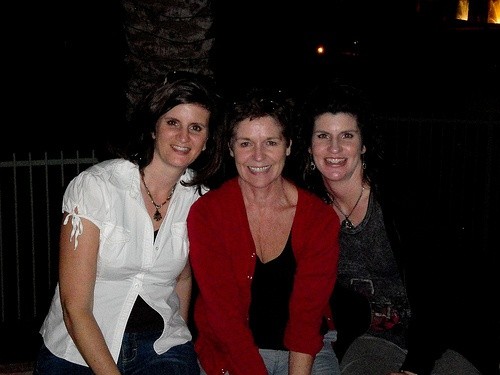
[39,80,223,375]
[296,101,481,375]
[187,98,342,375]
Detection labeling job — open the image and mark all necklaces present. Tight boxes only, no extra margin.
[140,168,177,221]
[331,188,363,231]
